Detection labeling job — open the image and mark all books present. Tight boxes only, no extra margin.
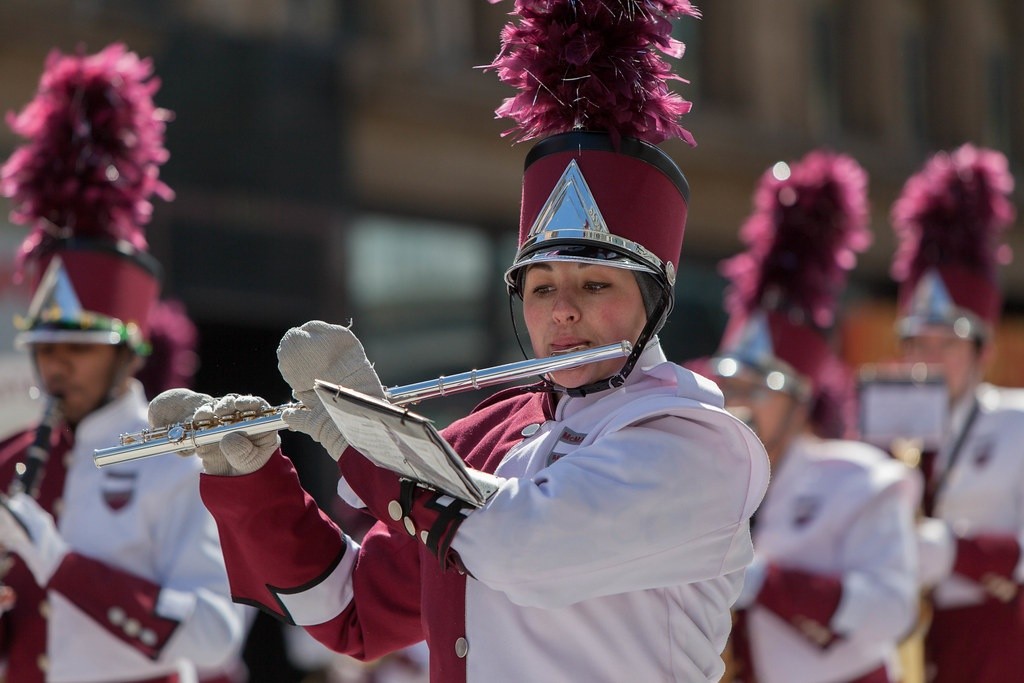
[312,377,489,512]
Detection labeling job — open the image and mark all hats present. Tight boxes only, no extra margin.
[893,253,998,340]
[12,234,163,353]
[708,299,838,402]
[504,132,691,318]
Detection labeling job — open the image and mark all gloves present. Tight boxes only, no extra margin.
[277,318,389,463]
[146,388,282,477]
[0,492,69,590]
[731,556,766,610]
[916,515,957,572]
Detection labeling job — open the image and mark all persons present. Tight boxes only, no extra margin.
[143,131,775,681]
[668,288,916,682]
[846,263,1024,683]
[0,227,262,683]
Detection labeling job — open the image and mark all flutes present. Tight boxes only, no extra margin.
[93,339,632,469]
